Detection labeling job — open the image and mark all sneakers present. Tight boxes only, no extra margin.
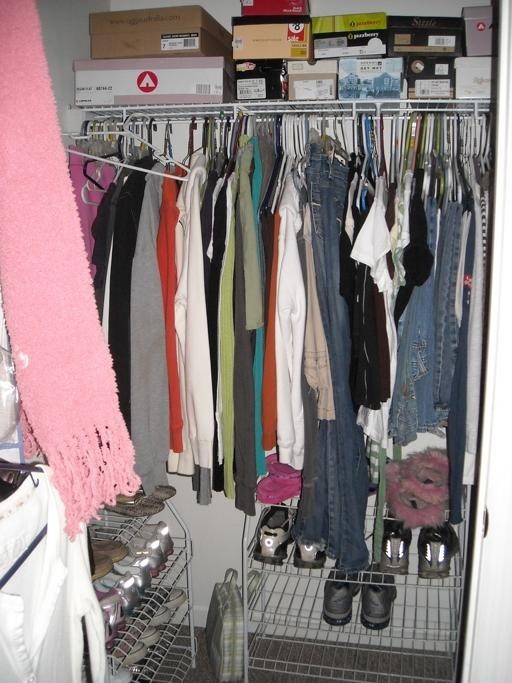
[379,514,412,574]
[90,485,185,683]
[360,565,396,629]
[418,521,456,579]
[323,558,361,625]
[253,504,326,568]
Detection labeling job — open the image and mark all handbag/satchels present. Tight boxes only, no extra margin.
[206,569,260,683]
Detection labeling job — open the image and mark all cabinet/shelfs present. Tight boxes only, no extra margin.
[85,479,200,680]
[240,495,466,683]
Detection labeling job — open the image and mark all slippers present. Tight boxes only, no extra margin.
[256,452,301,503]
[387,447,450,528]
